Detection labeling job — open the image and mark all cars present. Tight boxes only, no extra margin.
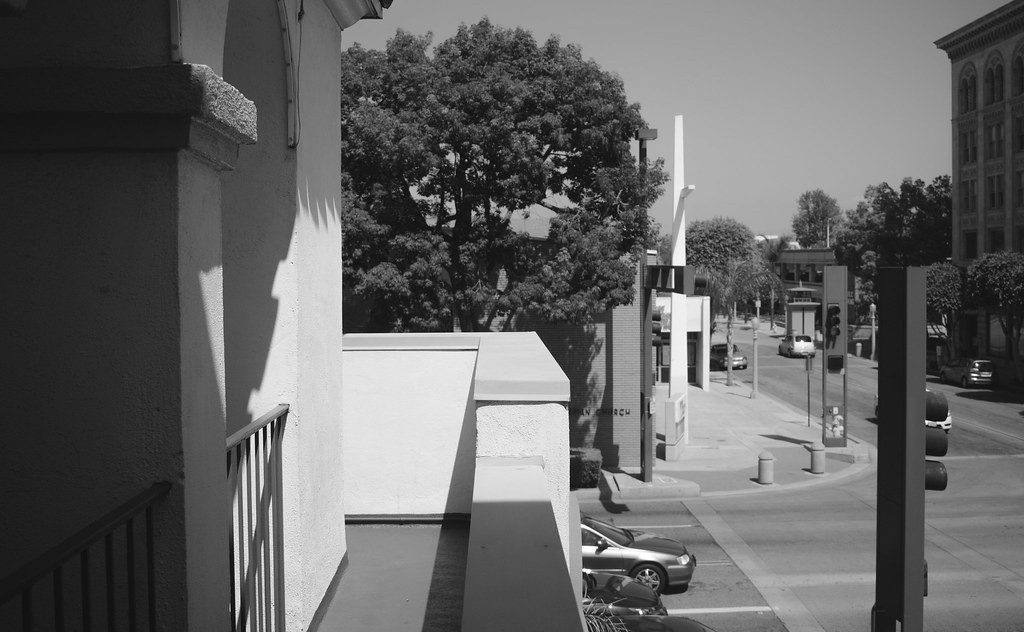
[710,344,748,369]
[579,516,697,596]
[578,568,669,617]
[778,335,816,357]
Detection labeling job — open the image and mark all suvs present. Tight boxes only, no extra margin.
[939,358,995,387]
